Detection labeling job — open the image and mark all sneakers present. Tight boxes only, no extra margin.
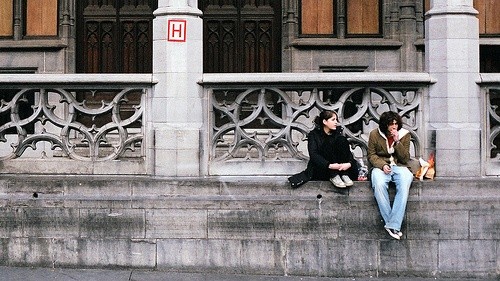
[341,175,353,186]
[384,225,403,240]
[330,175,346,188]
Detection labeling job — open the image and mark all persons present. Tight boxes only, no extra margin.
[367,111,414,239]
[287,109,359,188]
